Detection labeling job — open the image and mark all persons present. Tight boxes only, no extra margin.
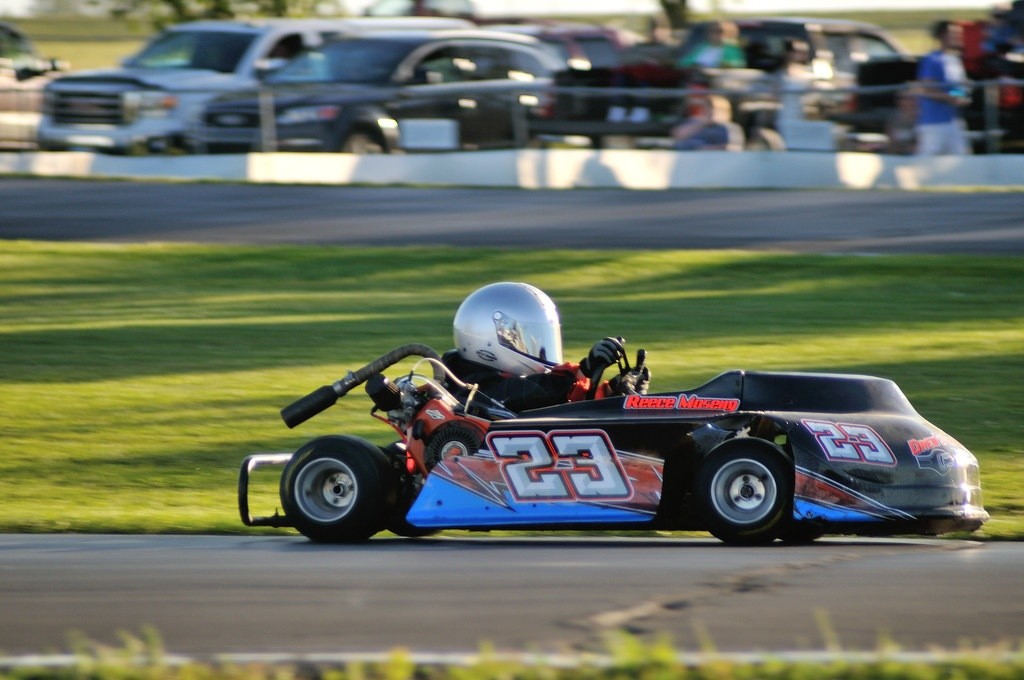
[627,0,1023,156]
[442,282,650,416]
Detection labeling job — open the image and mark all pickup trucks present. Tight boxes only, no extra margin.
[481,17,1024,146]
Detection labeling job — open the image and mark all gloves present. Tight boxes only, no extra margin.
[579,336,625,378]
[608,366,650,395]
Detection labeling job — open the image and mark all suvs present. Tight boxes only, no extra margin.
[35,18,477,154]
[198,31,569,152]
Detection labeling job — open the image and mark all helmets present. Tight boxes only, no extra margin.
[452,282,564,375]
